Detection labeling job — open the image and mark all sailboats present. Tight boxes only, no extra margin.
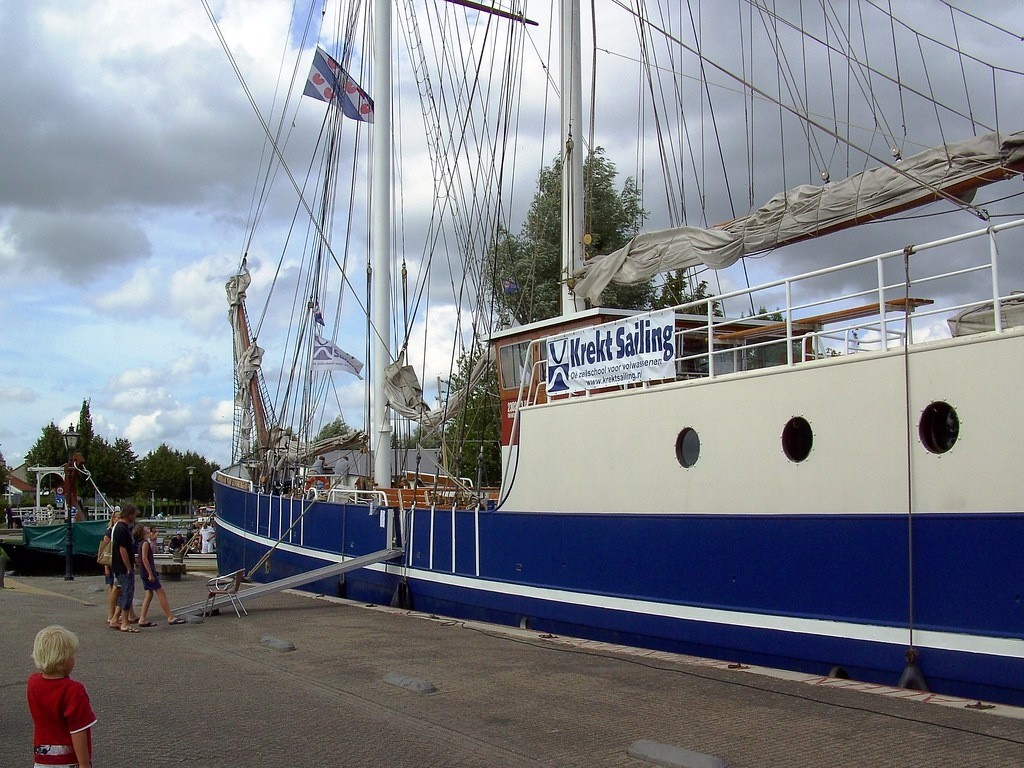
[207,1,1023,709]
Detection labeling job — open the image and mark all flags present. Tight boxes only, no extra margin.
[302,45,374,123]
[206,505,215,513]
[501,278,518,292]
[312,329,364,379]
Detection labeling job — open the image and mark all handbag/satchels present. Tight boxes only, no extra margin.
[97,541,113,566]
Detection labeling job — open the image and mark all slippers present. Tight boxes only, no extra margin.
[110,622,122,628]
[139,621,157,627]
[121,626,140,633]
[169,618,186,625]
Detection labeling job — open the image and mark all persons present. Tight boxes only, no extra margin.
[46,504,56,526]
[26,623,97,768]
[335,456,350,480]
[132,524,186,628]
[0,546,9,591]
[165,523,216,556]
[6,504,14,529]
[304,456,330,491]
[103,504,140,634]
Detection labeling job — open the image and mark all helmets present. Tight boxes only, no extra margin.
[176,531,182,535]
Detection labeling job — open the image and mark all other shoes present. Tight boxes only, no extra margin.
[107,616,122,623]
[128,617,140,623]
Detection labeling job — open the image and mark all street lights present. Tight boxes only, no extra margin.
[62,423,79,581]
[151,489,155,517]
[186,466,198,517]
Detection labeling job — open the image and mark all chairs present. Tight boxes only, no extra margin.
[203,568,249,619]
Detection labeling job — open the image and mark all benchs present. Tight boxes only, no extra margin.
[375,488,467,510]
[154,561,186,581]
[704,298,935,377]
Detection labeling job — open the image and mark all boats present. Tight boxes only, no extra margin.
[134,510,218,570]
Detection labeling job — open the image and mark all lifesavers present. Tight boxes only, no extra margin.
[306,476,330,490]
